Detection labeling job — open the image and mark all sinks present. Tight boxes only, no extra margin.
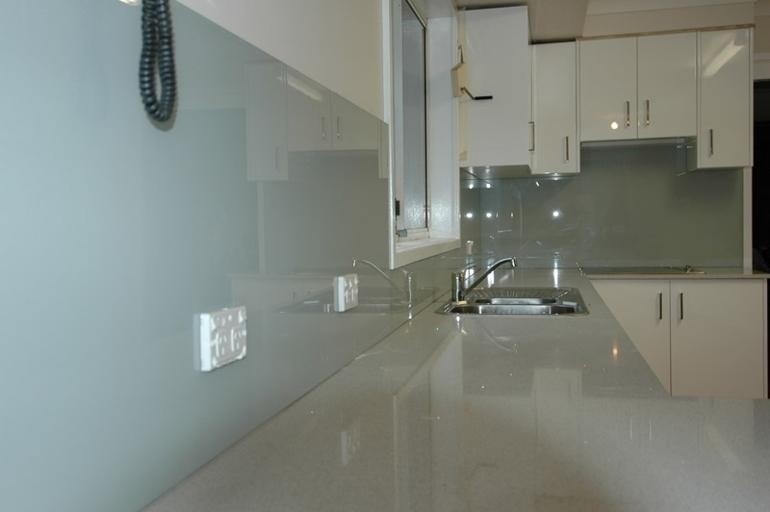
[321,282,439,298]
[273,300,410,314]
[490,297,559,306]
[434,301,576,315]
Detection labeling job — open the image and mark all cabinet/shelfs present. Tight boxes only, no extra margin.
[530,41,581,175]
[686,23,755,170]
[283,78,384,153]
[580,30,696,146]
[591,278,766,401]
[457,4,537,179]
[237,48,292,183]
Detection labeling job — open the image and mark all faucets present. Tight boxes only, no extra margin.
[352,256,417,305]
[451,256,520,301]
